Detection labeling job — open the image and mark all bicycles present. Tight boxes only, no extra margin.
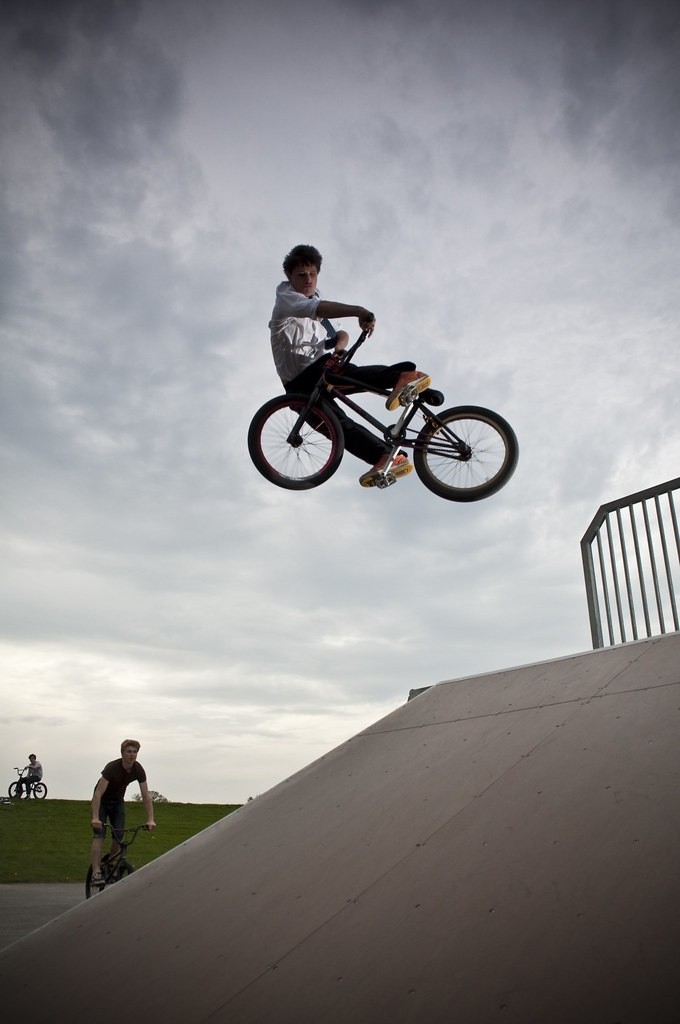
[84,822,155,899]
[7,767,47,799]
[246,313,520,503]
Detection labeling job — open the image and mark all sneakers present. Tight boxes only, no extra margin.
[359,454,413,487]
[385,370,430,412]
[91,871,106,885]
[107,863,117,877]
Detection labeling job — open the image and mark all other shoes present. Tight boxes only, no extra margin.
[23,796,31,800]
[13,795,17,798]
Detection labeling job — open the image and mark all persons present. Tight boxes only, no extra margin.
[270,245,432,489]
[12,754,43,799]
[91,739,157,886]
